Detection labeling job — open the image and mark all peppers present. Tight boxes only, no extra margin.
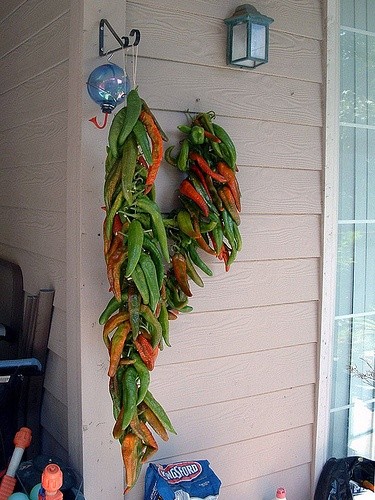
[161,111,242,320]
[98,86,175,496]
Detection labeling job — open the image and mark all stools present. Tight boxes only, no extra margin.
[14,454,77,499]
